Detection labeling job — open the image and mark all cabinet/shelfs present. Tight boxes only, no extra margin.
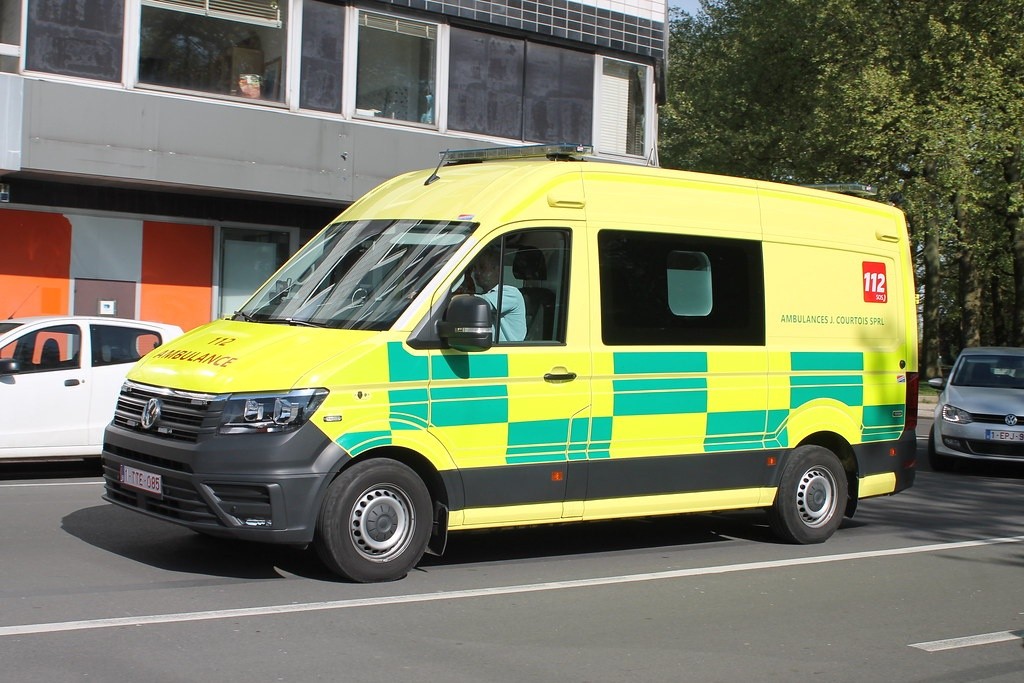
[192,47,264,99]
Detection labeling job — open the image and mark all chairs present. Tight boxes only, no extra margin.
[513,244,557,340]
[41,339,59,369]
[972,363,991,386]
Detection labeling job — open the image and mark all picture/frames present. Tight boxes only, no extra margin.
[262,57,282,100]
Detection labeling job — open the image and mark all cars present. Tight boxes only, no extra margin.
[927,346,1024,477]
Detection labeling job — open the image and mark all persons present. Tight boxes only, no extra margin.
[408,249,528,342]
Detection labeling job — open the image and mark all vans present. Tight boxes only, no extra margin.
[0,315,188,472]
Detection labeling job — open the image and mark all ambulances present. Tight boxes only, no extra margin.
[101,143,918,583]
